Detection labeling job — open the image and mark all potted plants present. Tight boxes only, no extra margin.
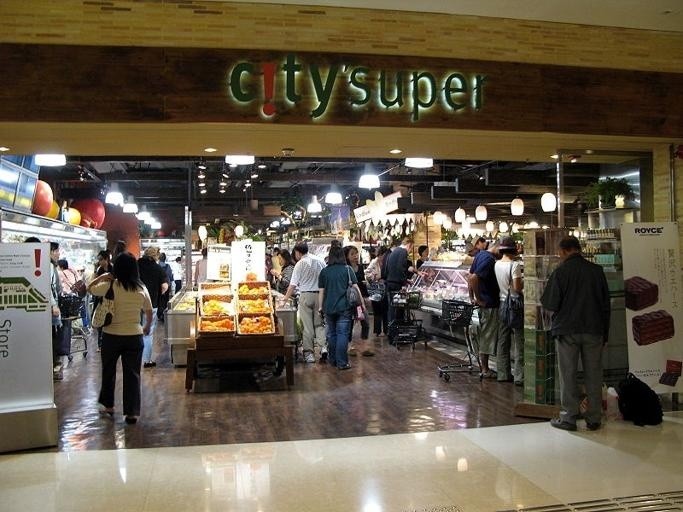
[585,176,637,208]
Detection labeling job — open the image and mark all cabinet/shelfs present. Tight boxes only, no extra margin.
[404,260,474,343]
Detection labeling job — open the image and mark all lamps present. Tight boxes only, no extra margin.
[433,191,557,228]
[305,158,434,213]
[104,181,151,221]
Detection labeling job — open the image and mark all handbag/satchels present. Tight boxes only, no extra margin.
[346,286,361,306]
[502,295,523,328]
[366,282,385,301]
[70,280,85,298]
[91,288,113,328]
[618,373,661,427]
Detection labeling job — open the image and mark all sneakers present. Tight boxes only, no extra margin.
[549,418,576,431]
[479,370,523,386]
[126,416,137,424]
[144,360,156,368]
[303,346,376,370]
[98,406,114,416]
[587,422,600,430]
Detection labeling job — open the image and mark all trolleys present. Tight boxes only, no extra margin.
[436,298,483,382]
[58,295,88,361]
[387,290,429,350]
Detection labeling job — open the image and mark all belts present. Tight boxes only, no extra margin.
[306,291,319,293]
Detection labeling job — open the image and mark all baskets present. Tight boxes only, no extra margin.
[441,300,473,326]
[198,281,275,335]
[389,292,422,309]
[58,296,81,318]
[389,319,422,341]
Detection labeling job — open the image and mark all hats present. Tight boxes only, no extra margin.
[465,235,515,254]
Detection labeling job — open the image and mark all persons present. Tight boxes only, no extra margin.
[192,247,207,287]
[467,236,489,257]
[23,235,185,382]
[536,235,612,432]
[86,252,154,425]
[267,238,431,371]
[466,239,503,378]
[492,236,525,388]
